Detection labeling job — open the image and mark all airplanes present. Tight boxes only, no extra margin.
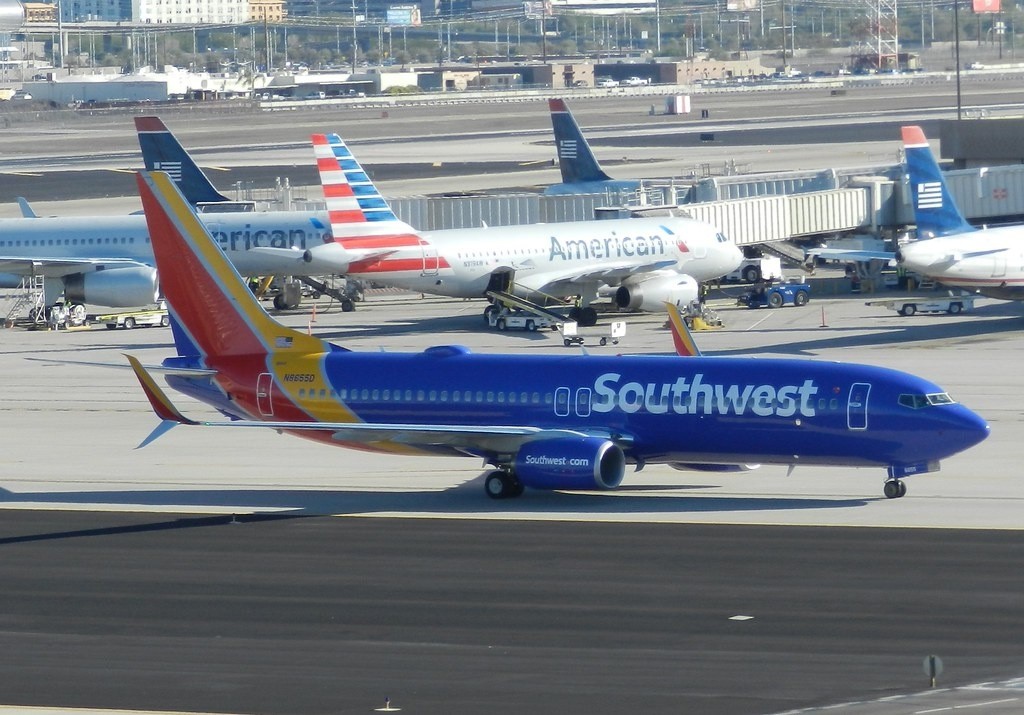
[0,97,745,347]
[123,167,992,501]
[804,127,1024,301]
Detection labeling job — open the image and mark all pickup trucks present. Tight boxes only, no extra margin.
[597,79,619,88]
[621,76,647,86]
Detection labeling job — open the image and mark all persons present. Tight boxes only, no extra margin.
[896,264,908,289]
[63,300,72,309]
[247,277,264,294]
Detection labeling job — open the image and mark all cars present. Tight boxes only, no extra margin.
[573,80,588,87]
[692,72,784,85]
